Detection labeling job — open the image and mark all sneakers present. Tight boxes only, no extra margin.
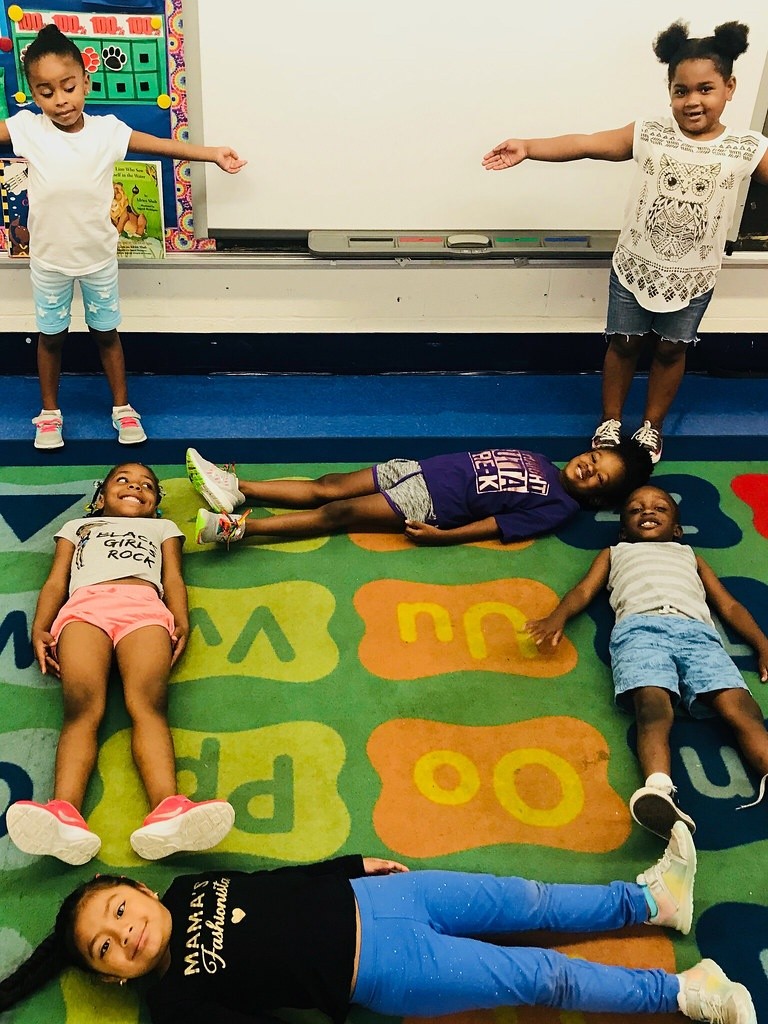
[590,416,623,451]
[195,507,254,551]
[676,956,756,1024]
[631,417,664,467]
[31,408,64,449]
[112,402,148,444]
[7,795,105,865]
[129,793,235,860]
[636,822,697,937]
[185,448,247,513]
[627,781,695,844]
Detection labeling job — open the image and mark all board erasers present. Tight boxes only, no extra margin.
[447,235,491,248]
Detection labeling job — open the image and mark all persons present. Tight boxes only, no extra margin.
[0,24,248,448]
[522,487,768,841]
[186,438,653,551]
[483,20,768,464]
[6,464,235,865]
[0,820,759,1024]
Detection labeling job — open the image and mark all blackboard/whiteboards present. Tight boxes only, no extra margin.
[180,0,768,260]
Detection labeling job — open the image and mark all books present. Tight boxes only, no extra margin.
[113,160,166,261]
[0,158,30,258]
